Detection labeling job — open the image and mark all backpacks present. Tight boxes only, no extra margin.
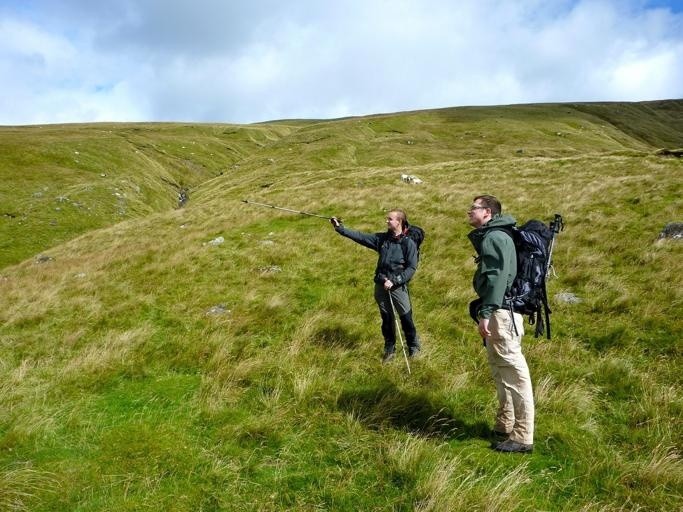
[375,225,425,270]
[484,226,549,315]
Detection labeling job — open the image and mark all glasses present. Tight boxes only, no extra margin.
[468,205,489,210]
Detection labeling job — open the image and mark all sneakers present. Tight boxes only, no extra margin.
[478,430,510,441]
[409,346,420,357]
[495,439,534,453]
[383,352,394,361]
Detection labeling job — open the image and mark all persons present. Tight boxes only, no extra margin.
[466,194,535,454]
[327,208,422,365]
